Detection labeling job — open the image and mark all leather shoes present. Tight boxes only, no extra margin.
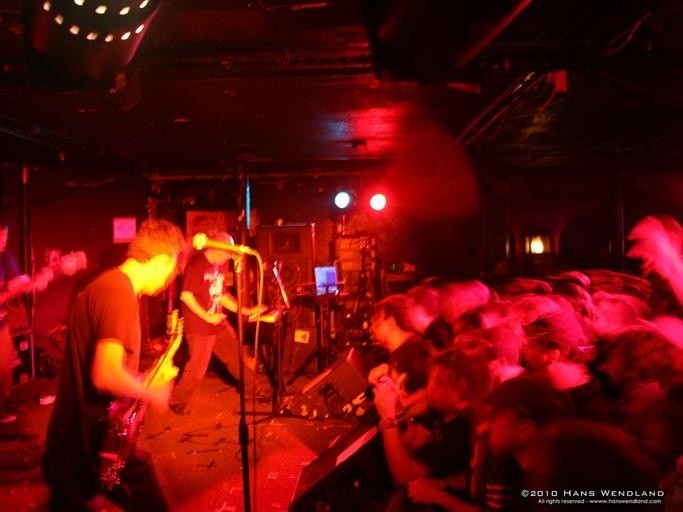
[168,403,190,414]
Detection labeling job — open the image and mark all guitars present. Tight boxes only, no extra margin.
[99,316,190,492]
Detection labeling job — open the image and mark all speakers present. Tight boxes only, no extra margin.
[303,348,367,419]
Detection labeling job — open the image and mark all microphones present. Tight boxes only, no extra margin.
[193,234,249,257]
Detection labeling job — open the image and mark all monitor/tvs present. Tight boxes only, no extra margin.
[313,265,337,295]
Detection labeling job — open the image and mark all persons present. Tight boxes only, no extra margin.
[2,218,683,512]
